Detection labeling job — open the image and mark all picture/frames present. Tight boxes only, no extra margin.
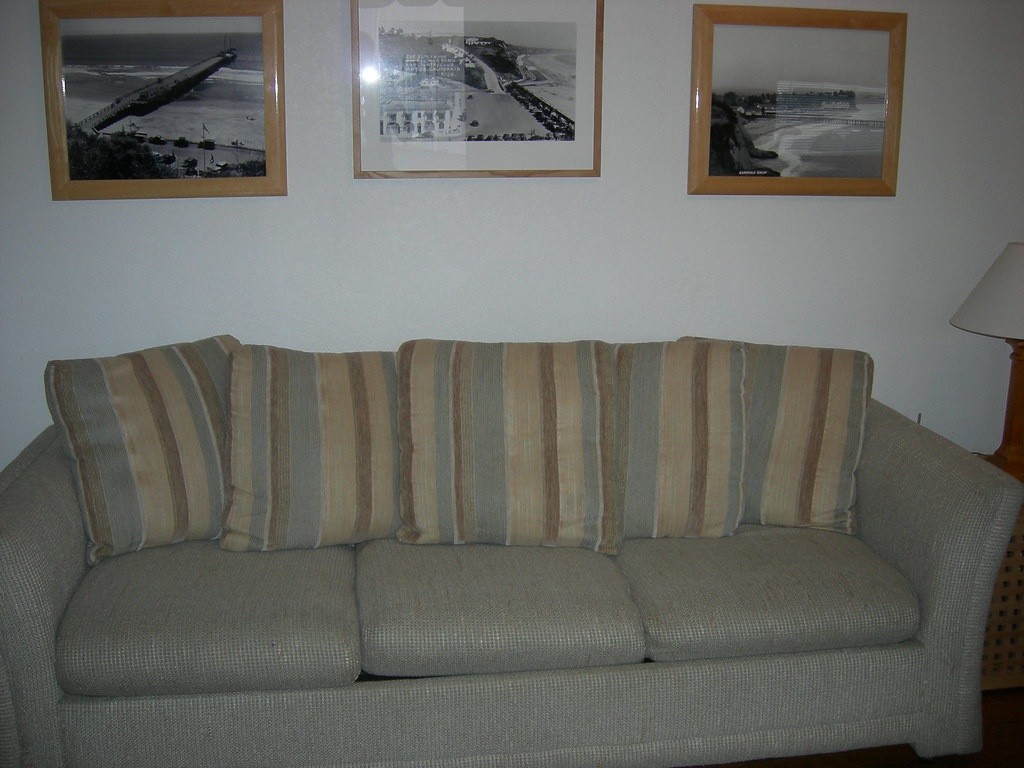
[688,4,907,197]
[39,0,290,201]
[352,0,602,180]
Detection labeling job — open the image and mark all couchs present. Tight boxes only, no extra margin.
[2,361,1023,768]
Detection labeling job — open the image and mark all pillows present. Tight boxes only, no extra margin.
[678,336,875,532]
[45,332,247,567]
[603,338,753,535]
[394,336,624,556]
[224,341,407,553]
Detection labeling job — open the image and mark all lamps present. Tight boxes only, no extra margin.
[950,242,1023,481]
